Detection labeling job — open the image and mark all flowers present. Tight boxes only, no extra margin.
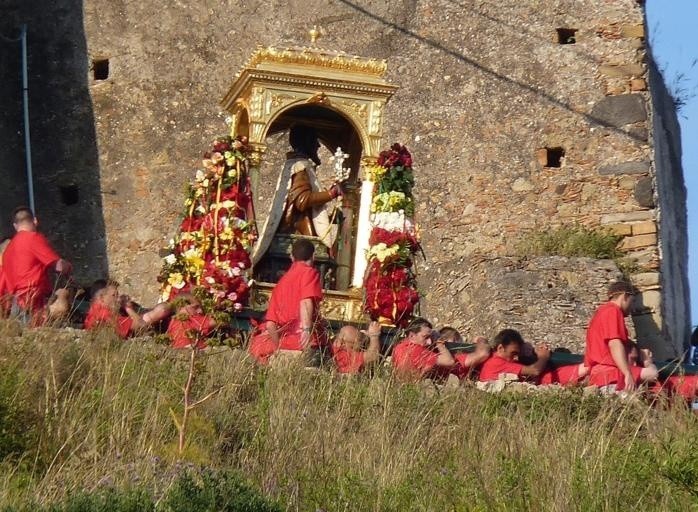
[363,141,424,327]
[157,136,259,325]
[319,145,352,243]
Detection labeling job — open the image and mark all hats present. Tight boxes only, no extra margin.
[609,282,632,297]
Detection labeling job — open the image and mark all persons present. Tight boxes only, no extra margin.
[265,238,322,367]
[3,206,72,327]
[276,122,349,237]
[81,281,230,349]
[329,318,589,388]
[0,238,12,319]
[585,281,696,407]
